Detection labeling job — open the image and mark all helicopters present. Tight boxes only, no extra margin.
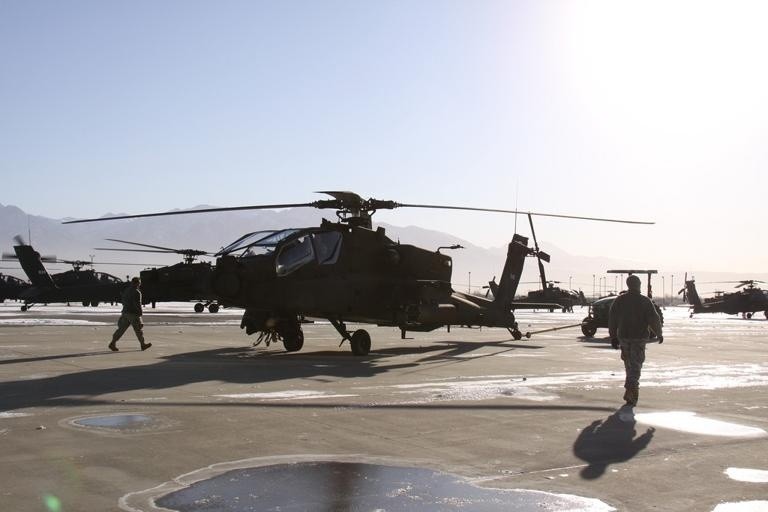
[677,272,768,322]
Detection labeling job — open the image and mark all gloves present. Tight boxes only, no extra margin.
[656,335,663,344]
[612,338,620,349]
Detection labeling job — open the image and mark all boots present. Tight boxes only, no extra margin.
[108,339,120,351]
[623,389,636,405]
[140,341,152,350]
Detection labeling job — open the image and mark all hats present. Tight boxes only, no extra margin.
[627,275,641,286]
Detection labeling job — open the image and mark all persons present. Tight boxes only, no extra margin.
[108,277,152,351]
[607,275,662,405]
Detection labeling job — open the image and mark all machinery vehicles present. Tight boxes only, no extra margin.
[580,269,664,343]
